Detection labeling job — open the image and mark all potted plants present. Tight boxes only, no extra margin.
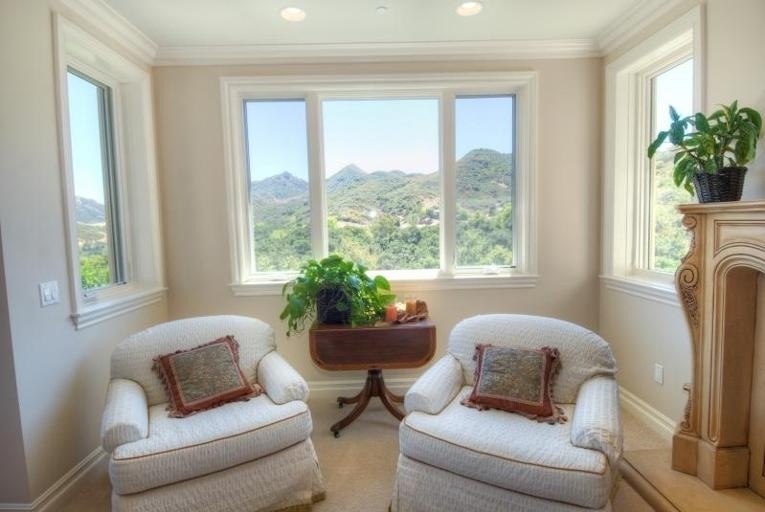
[278,249,398,338]
[647,99,763,206]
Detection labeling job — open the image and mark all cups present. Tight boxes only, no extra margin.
[384,307,397,320]
[406,300,417,314]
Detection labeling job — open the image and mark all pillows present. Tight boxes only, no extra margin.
[149,334,264,419]
[459,339,568,426]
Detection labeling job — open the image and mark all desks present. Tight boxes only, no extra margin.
[307,317,438,438]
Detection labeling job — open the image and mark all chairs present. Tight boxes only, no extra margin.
[390,313,624,512]
[100,314,327,512]
